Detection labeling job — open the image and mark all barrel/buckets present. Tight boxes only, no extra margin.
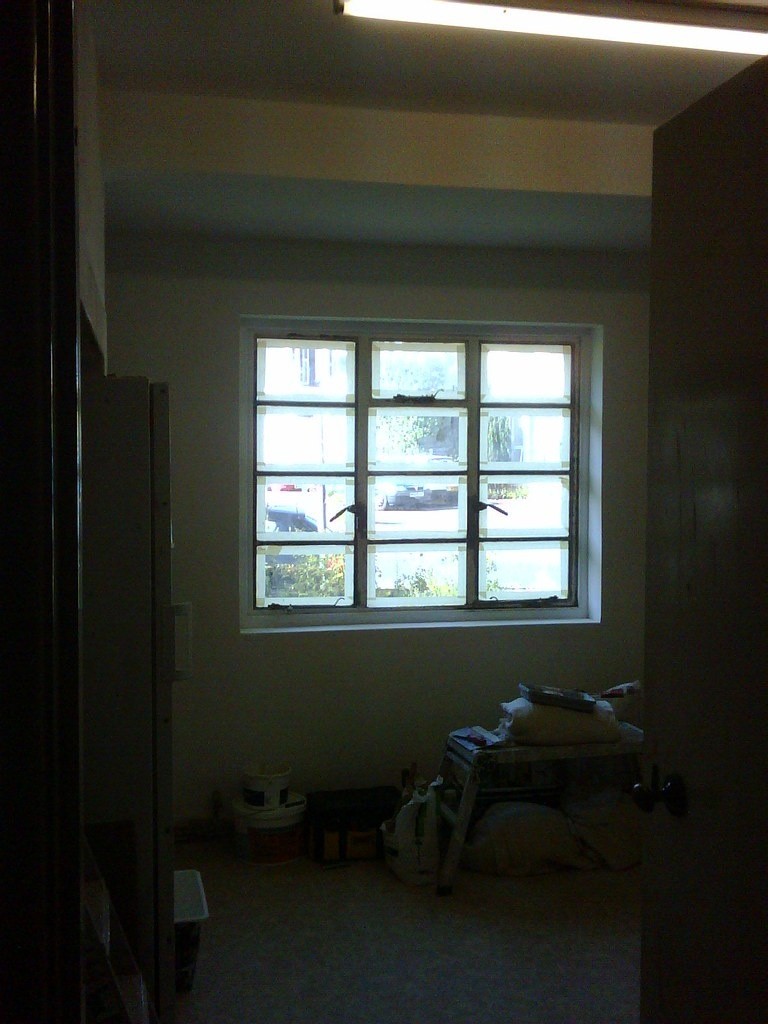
[231,791,308,868]
[240,762,293,809]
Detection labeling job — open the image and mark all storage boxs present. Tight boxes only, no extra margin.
[173,868,208,991]
[307,784,402,866]
